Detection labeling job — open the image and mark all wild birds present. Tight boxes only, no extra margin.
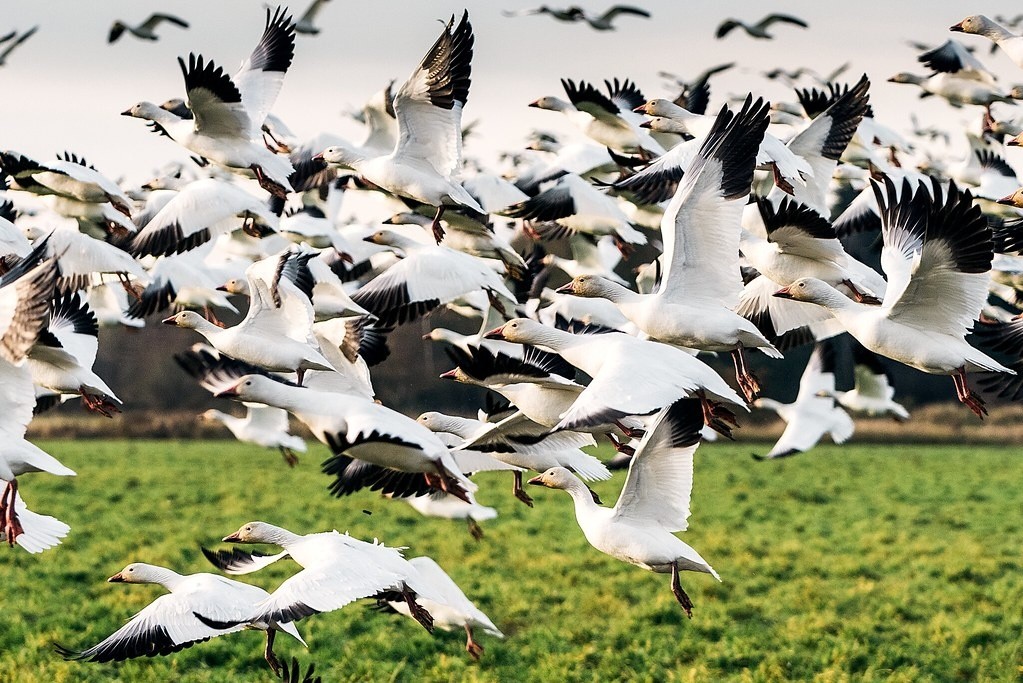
[1,0,1023,683]
